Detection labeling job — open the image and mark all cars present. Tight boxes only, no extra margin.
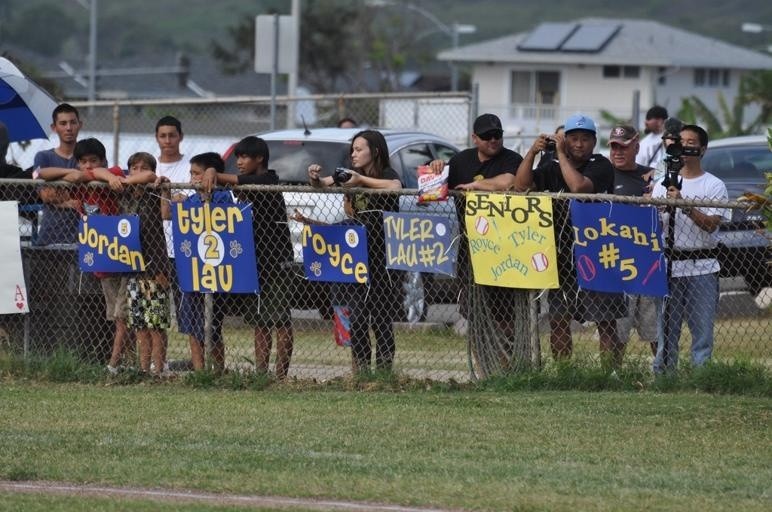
[699,136,772,295]
[221,123,461,322]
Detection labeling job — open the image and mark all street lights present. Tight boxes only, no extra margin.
[741,21,772,34]
[406,3,461,90]
[411,22,477,51]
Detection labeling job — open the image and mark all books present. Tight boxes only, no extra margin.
[418,165,449,201]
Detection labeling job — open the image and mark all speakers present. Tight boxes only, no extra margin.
[538,140,560,170]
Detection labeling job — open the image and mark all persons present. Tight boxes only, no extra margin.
[56,139,133,383]
[535,126,572,164]
[96,150,170,382]
[0,122,25,221]
[32,100,91,252]
[306,130,404,370]
[203,135,294,380]
[152,152,234,375]
[652,124,734,375]
[429,113,524,374]
[148,115,197,280]
[335,119,360,128]
[515,115,618,372]
[634,106,674,173]
[603,124,661,358]
[292,195,354,228]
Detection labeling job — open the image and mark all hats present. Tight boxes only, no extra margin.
[564,114,596,134]
[474,114,502,135]
[607,125,640,147]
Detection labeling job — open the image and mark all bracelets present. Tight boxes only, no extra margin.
[88,169,97,181]
[681,206,692,214]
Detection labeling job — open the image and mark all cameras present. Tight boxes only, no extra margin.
[334,167,352,182]
[661,118,700,157]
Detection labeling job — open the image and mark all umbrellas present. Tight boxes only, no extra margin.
[0,58,64,141]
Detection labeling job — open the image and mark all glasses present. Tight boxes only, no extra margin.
[475,130,503,141]
